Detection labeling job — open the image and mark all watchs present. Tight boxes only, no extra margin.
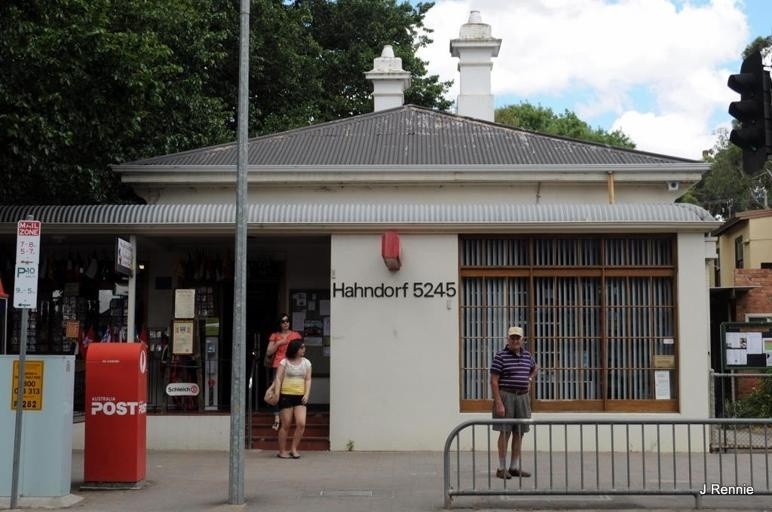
[529,377,532,381]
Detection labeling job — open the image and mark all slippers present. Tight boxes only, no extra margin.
[277,452,300,459]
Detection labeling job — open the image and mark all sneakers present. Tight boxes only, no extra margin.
[497,469,511,478]
[509,468,530,476]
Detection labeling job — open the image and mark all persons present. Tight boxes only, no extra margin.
[266,312,302,432]
[274,338,313,458]
[489,327,538,479]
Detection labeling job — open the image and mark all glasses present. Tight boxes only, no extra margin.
[281,319,289,323]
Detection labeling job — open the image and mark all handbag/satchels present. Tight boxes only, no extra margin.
[264,384,278,405]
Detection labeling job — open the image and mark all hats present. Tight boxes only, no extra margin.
[508,326,523,338]
